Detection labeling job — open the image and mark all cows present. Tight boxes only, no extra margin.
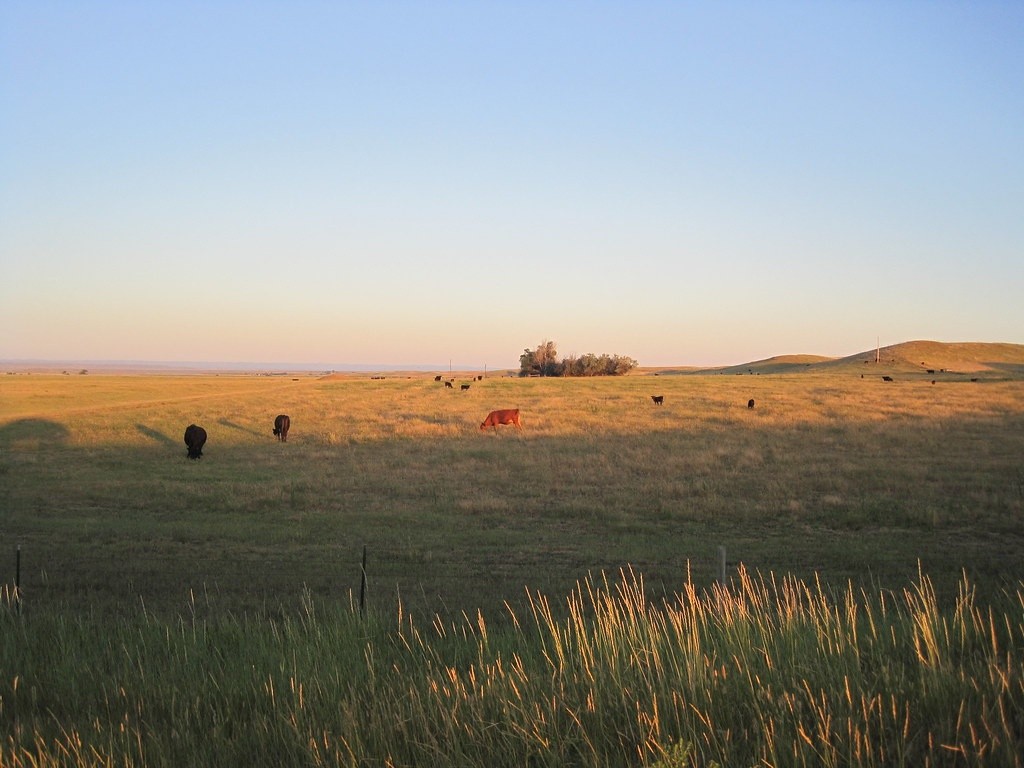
[184,424,207,462]
[747,398,755,409]
[650,395,664,406]
[480,408,523,437]
[272,414,290,442]
[434,375,483,391]
